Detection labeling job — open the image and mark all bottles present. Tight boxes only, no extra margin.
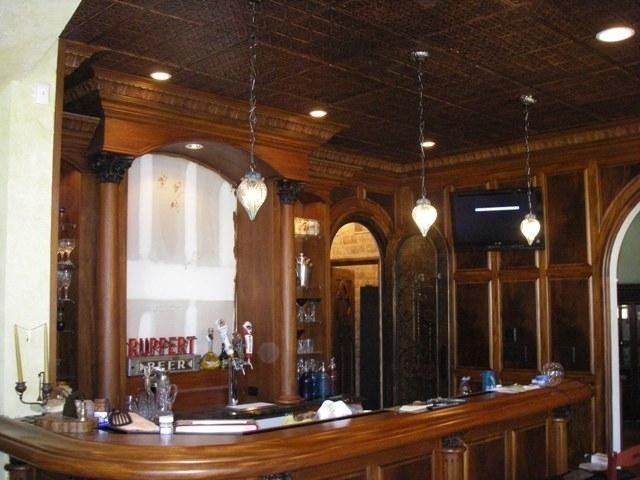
[218,342,230,369]
[199,334,220,370]
[138,365,178,424]
[296,356,339,403]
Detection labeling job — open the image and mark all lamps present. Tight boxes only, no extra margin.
[519,93,542,246]
[409,50,438,238]
[233,0,269,223]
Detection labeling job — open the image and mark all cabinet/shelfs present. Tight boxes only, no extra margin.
[236,176,334,406]
[56,145,97,401]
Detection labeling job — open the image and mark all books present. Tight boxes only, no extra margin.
[175,418,257,434]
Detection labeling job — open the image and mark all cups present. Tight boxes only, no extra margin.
[158,410,174,438]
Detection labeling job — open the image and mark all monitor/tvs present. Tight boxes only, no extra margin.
[449,186,545,251]
